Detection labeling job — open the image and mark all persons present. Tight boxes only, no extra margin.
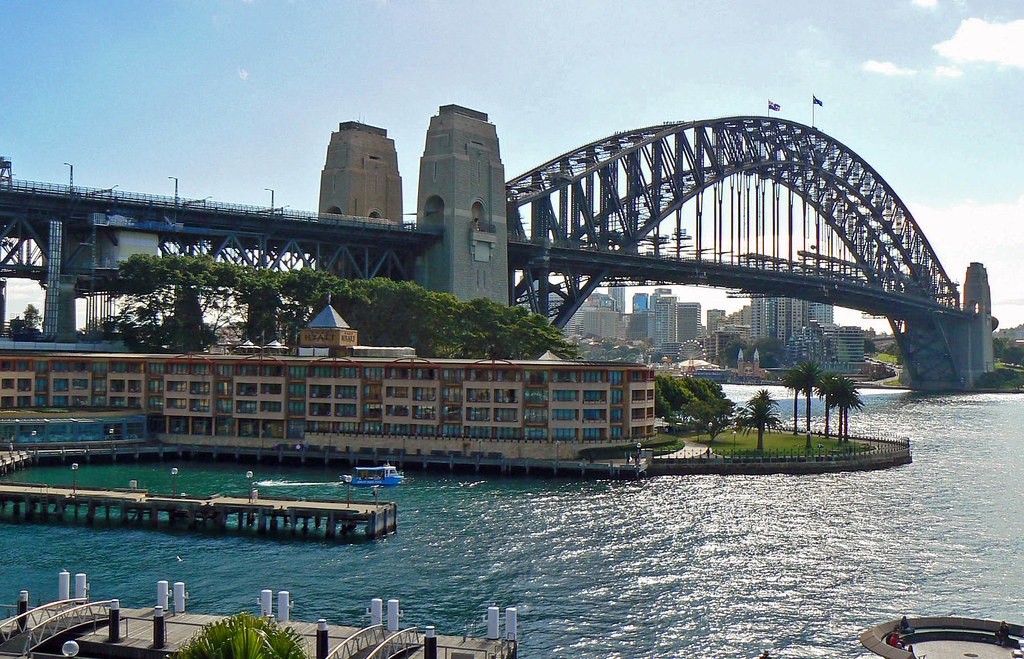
[360,472,367,479]
[995,621,1009,648]
[9,438,14,454]
[886,616,918,659]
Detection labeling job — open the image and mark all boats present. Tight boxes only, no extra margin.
[341,459,415,486]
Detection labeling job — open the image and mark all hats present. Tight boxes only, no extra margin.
[1002,621,1007,624]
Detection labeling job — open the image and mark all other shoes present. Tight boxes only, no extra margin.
[995,641,1000,645]
[1002,641,1005,645]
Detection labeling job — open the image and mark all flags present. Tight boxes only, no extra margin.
[768,99,780,111]
[813,95,823,107]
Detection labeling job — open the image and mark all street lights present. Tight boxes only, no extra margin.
[63,163,74,195]
[71,463,78,494]
[169,177,178,204]
[171,468,178,499]
[265,188,274,211]
[246,470,253,503]
[344,474,352,509]
[203,196,212,209]
